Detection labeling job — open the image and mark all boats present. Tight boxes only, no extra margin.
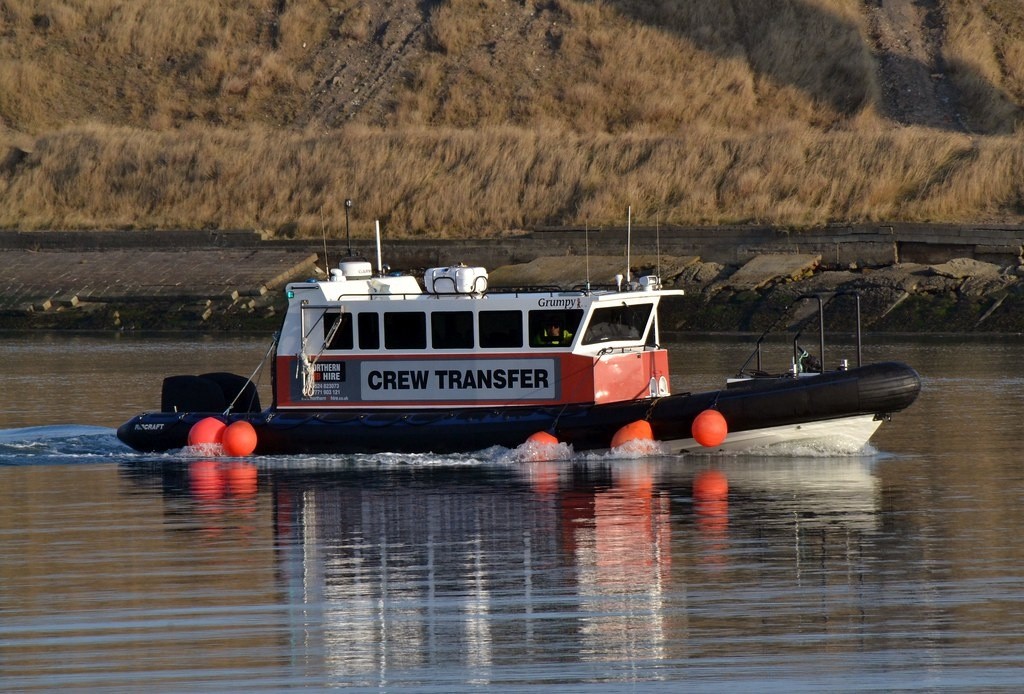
[117,198,923,458]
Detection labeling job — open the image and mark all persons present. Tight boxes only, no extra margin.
[539,319,573,347]
